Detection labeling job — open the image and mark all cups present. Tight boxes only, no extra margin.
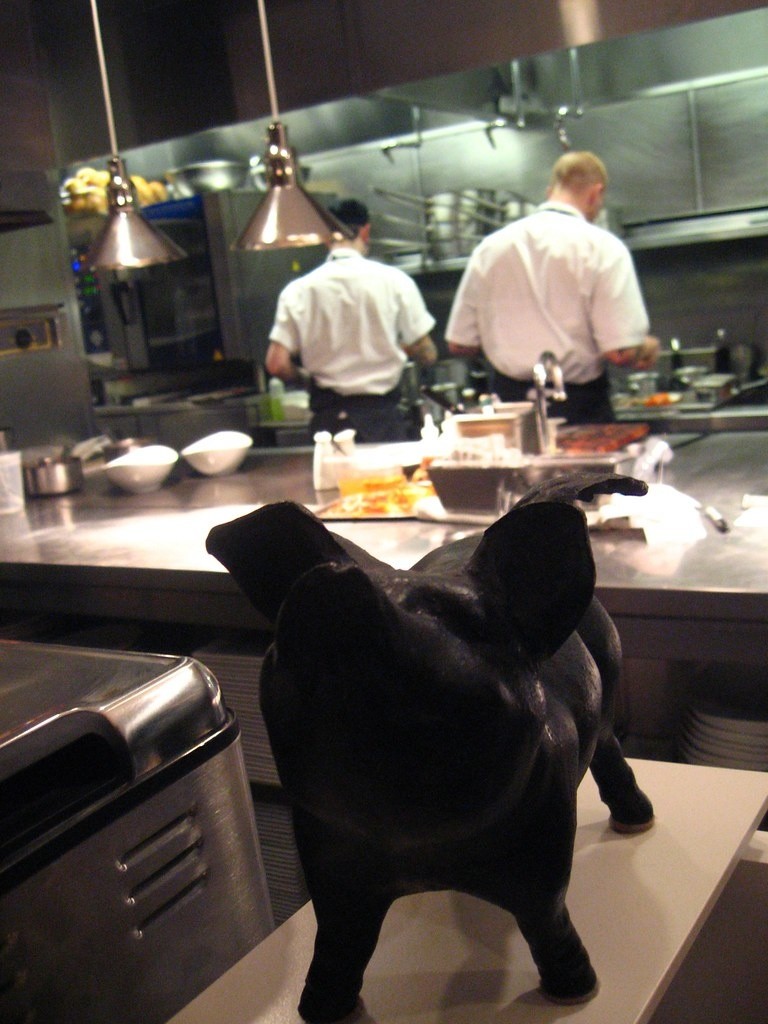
[0,450,26,515]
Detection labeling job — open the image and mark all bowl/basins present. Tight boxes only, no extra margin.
[103,445,180,494]
[26,457,83,495]
[181,430,254,477]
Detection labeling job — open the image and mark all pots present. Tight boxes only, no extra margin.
[364,182,537,256]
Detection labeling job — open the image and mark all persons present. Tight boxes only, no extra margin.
[443,152,660,426]
[265,198,439,445]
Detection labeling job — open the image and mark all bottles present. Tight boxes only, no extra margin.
[269,377,283,421]
[313,431,335,490]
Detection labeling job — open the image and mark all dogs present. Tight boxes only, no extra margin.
[204,471,654,1024]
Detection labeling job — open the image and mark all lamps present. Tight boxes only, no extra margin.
[231,0,357,252]
[87,0,187,272]
